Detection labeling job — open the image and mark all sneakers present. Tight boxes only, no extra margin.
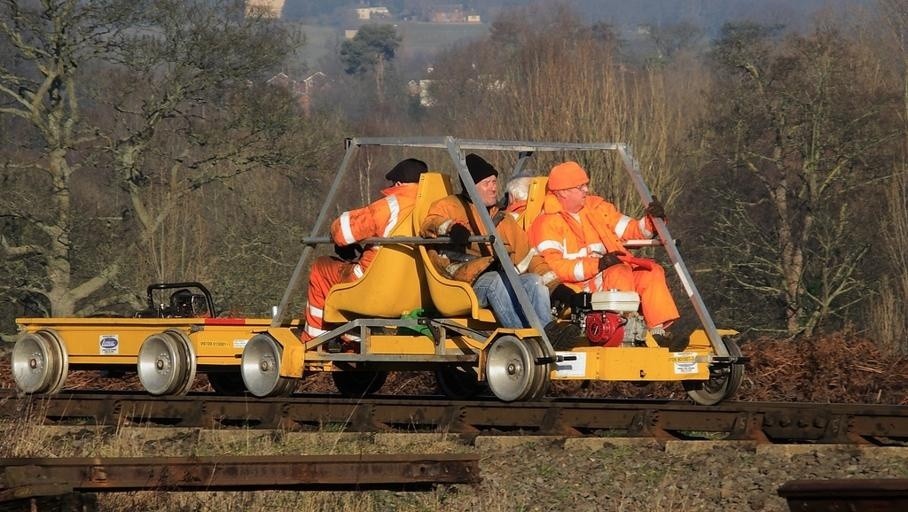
[325,336,342,352]
[653,314,700,352]
[544,320,580,351]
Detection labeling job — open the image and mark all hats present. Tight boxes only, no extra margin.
[508,156,540,182]
[386,159,427,182]
[458,153,498,189]
[547,161,590,191]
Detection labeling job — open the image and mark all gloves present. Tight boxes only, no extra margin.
[450,223,470,254]
[645,195,666,218]
[550,284,576,314]
[598,252,626,271]
[335,243,363,262]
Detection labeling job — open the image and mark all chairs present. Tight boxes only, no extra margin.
[323,172,582,323]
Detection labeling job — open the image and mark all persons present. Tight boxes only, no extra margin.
[300,159,428,353]
[530,161,681,347]
[418,153,580,351]
[505,177,529,215]
